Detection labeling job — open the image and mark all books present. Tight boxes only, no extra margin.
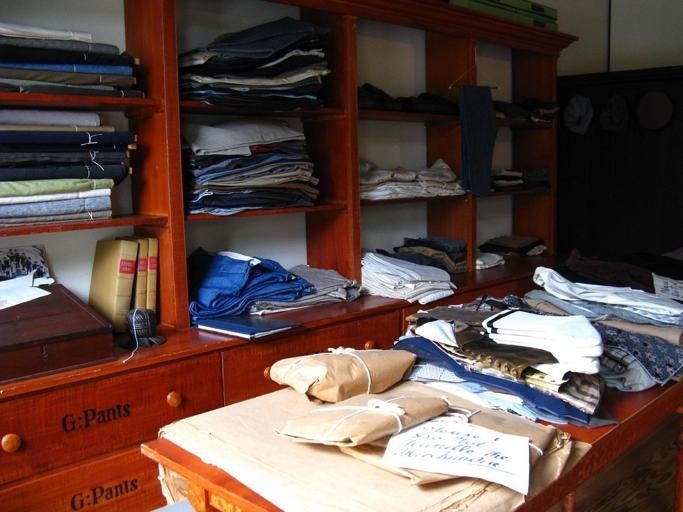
[87,234,158,333]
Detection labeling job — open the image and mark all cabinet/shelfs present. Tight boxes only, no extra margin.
[0,2,577,511]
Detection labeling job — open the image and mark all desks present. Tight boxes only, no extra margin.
[141,369,683,511]
